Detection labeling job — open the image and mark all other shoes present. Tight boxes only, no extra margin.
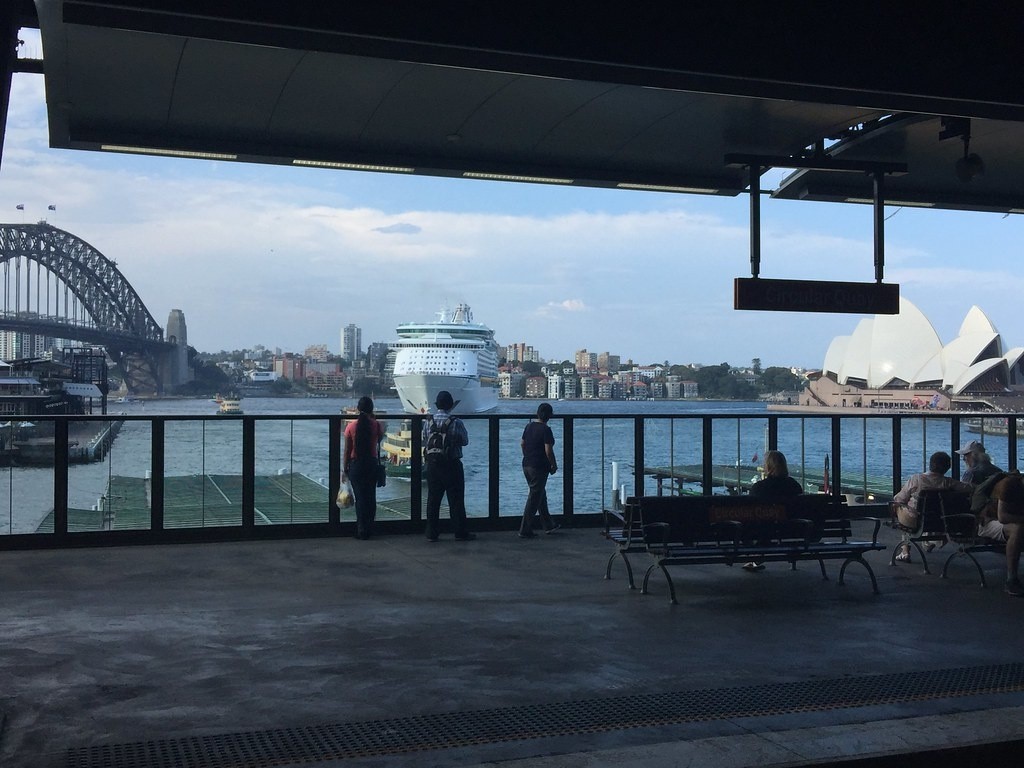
[518,532,539,537]
[425,535,441,541]
[545,522,562,533]
[895,551,911,562]
[357,531,370,539]
[1003,580,1023,595]
[922,542,936,552]
[455,532,477,540]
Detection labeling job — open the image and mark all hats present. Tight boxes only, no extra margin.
[434,391,453,409]
[954,439,986,454]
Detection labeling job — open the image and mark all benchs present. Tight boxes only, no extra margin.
[882,489,963,575]
[938,490,1024,588]
[639,497,887,605]
[600,494,852,588]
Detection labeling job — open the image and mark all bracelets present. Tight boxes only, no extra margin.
[343,469,348,472]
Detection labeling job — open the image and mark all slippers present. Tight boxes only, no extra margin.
[741,562,766,571]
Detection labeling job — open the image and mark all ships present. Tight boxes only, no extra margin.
[391,302,501,417]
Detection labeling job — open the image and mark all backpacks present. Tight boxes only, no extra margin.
[422,416,457,465]
[970,470,1023,513]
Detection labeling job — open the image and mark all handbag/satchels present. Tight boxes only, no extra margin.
[376,464,386,488]
[336,480,354,509]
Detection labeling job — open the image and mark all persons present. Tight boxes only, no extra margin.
[422,391,476,543]
[341,397,385,539]
[742,450,802,570]
[893,440,1024,595]
[518,403,562,538]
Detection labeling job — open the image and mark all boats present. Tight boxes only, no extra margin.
[215,400,244,417]
[379,419,427,479]
[336,405,388,434]
